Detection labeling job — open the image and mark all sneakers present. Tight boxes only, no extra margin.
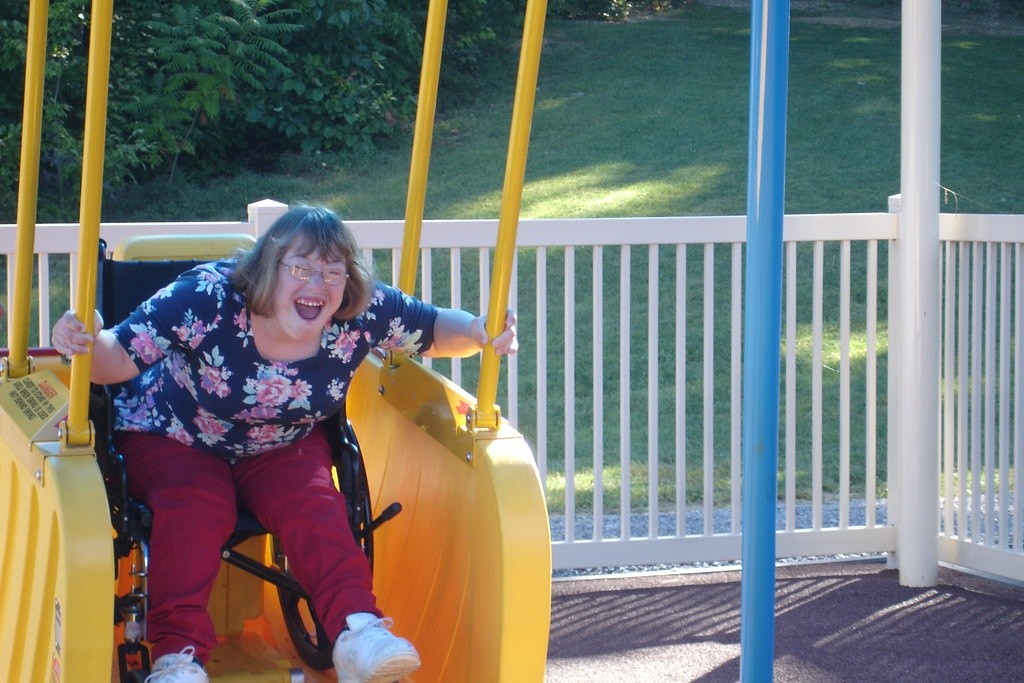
[332,612,421,682]
[143,646,208,683]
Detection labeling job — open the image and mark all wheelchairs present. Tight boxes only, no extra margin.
[88,238,401,683]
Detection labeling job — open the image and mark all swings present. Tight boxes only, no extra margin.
[0,0,548,683]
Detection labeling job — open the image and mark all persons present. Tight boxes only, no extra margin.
[49,205,519,683]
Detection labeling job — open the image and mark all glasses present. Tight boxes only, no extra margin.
[280,260,350,286]
